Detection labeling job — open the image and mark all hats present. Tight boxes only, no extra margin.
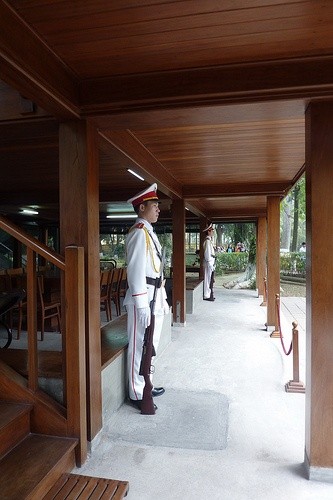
[203,223,214,232]
[127,183,158,207]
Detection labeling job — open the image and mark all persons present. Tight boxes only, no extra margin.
[201,221,217,302]
[299,242,306,252]
[122,183,167,412]
[217,240,247,252]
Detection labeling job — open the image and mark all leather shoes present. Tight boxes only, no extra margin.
[151,387,165,396]
[130,399,158,410]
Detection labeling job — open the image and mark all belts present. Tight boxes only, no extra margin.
[146,276,162,288]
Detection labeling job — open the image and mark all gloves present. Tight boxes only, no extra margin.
[164,299,169,315]
[207,264,215,271]
[137,306,151,328]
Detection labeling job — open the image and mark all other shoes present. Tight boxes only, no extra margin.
[204,298,215,301]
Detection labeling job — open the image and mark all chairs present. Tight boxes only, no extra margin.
[0,268,128,341]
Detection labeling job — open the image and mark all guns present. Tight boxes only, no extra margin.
[137,245,161,415]
[206,253,219,301]
